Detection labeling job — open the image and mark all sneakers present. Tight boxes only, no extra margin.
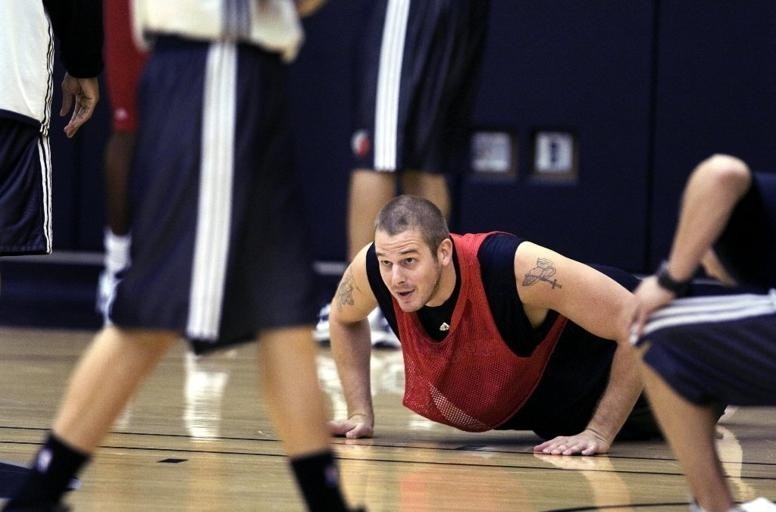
[314,303,402,351]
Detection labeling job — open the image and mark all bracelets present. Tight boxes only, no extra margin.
[656,260,688,291]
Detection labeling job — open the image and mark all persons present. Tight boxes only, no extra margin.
[319,191,670,454]
[93,0,145,316]
[1,1,369,512]
[0,0,103,258]
[618,149,776,510]
[312,0,490,351]
